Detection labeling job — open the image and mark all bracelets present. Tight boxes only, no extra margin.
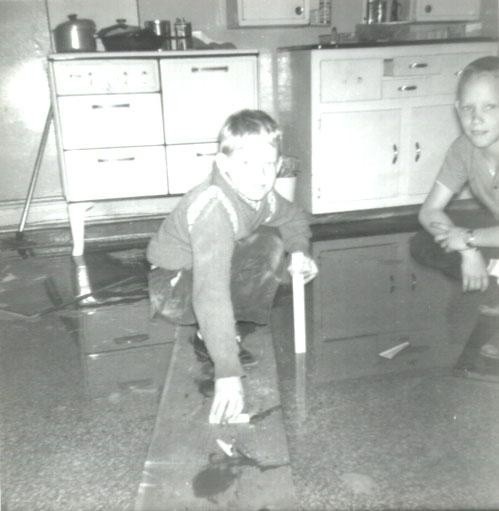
[464,224,478,250]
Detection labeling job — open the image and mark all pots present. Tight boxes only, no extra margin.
[91,17,142,54]
[174,17,193,38]
[50,14,98,54]
[144,20,172,40]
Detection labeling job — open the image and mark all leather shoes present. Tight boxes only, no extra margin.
[479,333,499,361]
[190,327,258,370]
[477,296,499,316]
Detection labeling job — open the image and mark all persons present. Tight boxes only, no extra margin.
[141,106,318,426]
[403,54,499,300]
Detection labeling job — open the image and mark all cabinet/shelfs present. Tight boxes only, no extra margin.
[158,59,263,146]
[165,141,222,197]
[56,97,167,151]
[62,151,168,200]
[53,61,161,94]
[288,45,499,216]
